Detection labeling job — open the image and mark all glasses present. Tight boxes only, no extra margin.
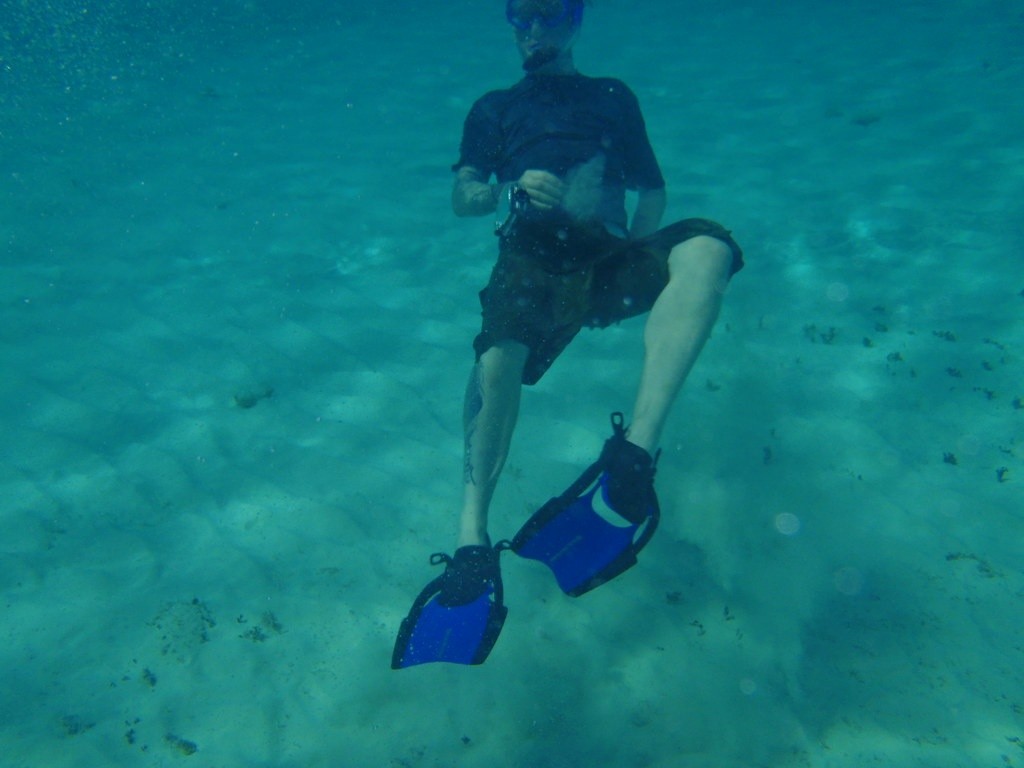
[505,0,586,29]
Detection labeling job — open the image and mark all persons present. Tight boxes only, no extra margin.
[390,0,744,673]
